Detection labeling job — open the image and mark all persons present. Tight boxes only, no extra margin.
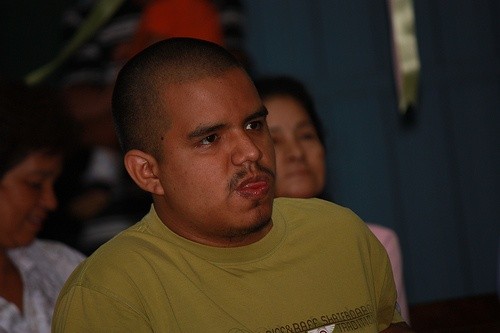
[0,0,407,333]
